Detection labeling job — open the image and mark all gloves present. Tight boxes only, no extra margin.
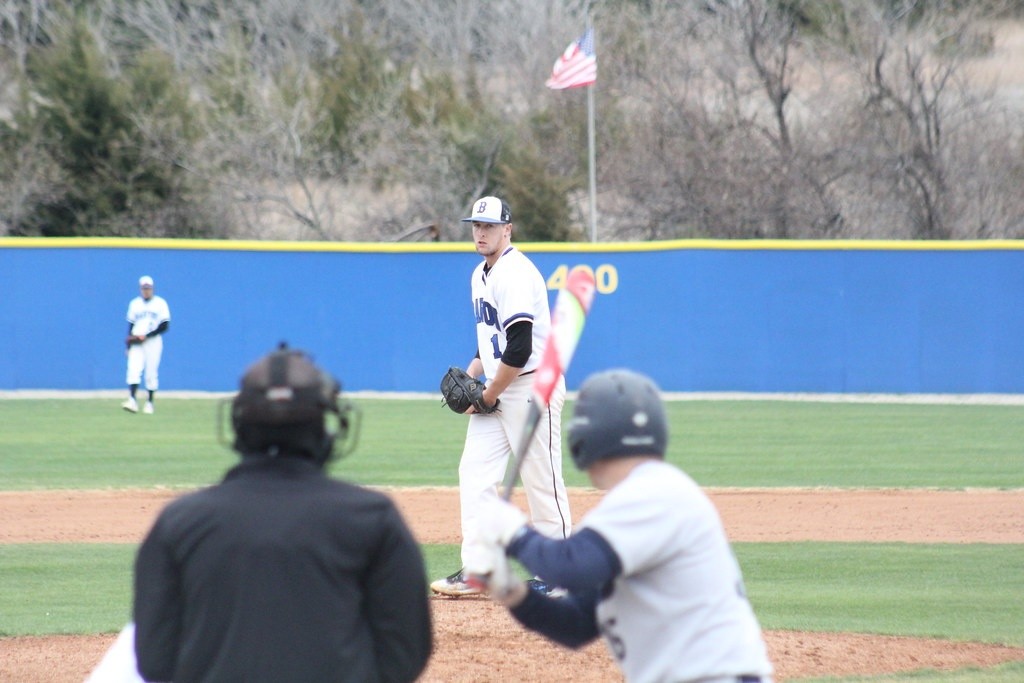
[472,488,529,547]
[462,526,515,603]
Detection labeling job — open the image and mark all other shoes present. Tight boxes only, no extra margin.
[123,399,138,413]
[142,401,153,414]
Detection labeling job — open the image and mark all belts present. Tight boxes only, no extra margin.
[518,369,537,377]
[736,676,759,683]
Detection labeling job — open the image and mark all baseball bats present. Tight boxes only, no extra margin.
[500,264,596,505]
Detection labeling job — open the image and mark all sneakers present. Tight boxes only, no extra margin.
[430,568,491,597]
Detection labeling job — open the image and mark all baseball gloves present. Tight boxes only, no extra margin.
[439,366,501,416]
[125,335,144,344]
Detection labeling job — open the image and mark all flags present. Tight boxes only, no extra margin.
[545,26,598,90]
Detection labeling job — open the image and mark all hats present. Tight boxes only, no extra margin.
[139,275,154,288]
[461,196,512,224]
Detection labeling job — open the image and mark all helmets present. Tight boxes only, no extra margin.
[232,351,340,429]
[567,369,667,468]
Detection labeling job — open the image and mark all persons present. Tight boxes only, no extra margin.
[429,196,569,598]
[121,275,171,415]
[132,350,432,683]
[483,369,775,683]
